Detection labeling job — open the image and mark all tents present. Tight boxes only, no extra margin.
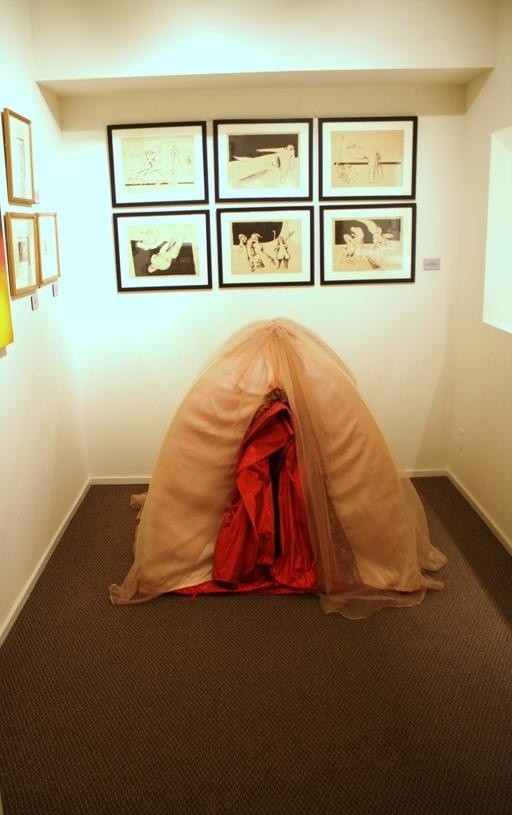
[108,317,449,623]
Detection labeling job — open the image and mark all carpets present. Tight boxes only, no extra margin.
[2,474,511,813]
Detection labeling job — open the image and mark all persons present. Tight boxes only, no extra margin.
[237,231,290,273]
[131,240,185,274]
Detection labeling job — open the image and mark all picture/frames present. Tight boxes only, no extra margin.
[2,210,43,301]
[214,116,316,205]
[316,112,420,201]
[103,119,212,208]
[36,211,64,286]
[0,109,39,207]
[112,211,215,294]
[319,202,417,288]
[214,204,317,292]
